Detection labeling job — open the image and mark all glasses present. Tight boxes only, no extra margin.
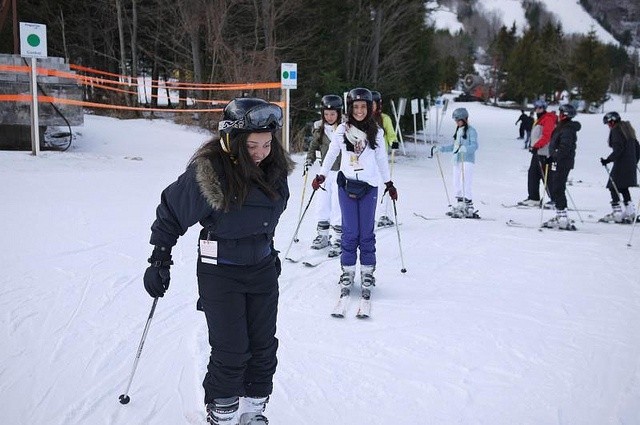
[535,106,543,109]
[218,103,283,131]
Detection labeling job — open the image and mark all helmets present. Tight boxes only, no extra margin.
[218,98,275,134]
[320,95,342,109]
[534,100,547,108]
[603,112,620,124]
[559,105,575,117]
[371,91,382,100]
[452,108,468,120]
[347,88,372,105]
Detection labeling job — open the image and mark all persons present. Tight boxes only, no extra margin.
[437,107,478,218]
[517,99,559,208]
[515,108,528,139]
[301,94,344,258]
[311,88,398,300]
[371,89,399,155]
[541,105,582,231]
[599,111,640,224]
[143,98,297,424]
[524,110,535,151]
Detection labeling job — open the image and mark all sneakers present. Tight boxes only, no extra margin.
[206,396,238,425]
[378,216,394,226]
[545,209,570,229]
[360,264,375,289]
[311,221,330,249]
[447,200,474,217]
[518,198,541,207]
[238,396,270,424]
[328,226,342,257]
[544,200,556,208]
[338,264,356,288]
[603,205,624,222]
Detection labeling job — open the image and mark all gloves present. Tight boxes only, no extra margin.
[457,146,467,152]
[312,175,325,190]
[144,251,173,297]
[383,181,397,201]
[302,159,312,176]
[601,158,610,166]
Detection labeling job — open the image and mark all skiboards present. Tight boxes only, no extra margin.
[583,215,640,224]
[413,211,498,220]
[502,202,596,212]
[567,177,590,187]
[286,235,343,266]
[507,220,591,231]
[331,288,372,319]
[331,220,404,232]
